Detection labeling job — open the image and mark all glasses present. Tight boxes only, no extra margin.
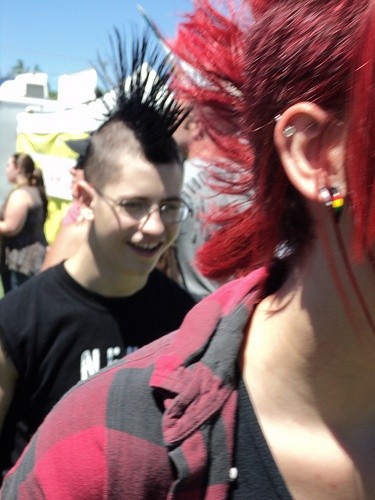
[91,185,194,224]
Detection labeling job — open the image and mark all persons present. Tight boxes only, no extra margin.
[0,0,375,500]
[39,129,92,275]
[0,152,48,296]
[154,85,269,304]
[0,29,196,489]
